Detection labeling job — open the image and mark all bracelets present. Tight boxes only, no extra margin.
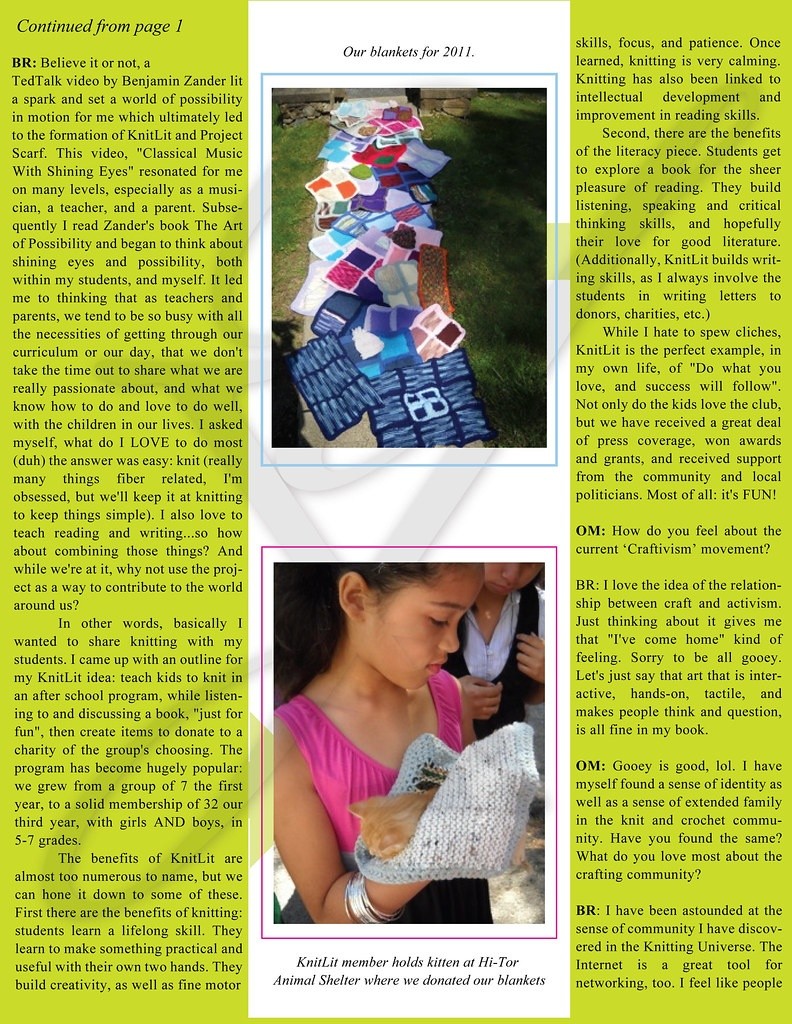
[345,870,403,923]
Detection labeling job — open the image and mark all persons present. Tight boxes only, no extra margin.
[441,563,545,742]
[274,563,493,923]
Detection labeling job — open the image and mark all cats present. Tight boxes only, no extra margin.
[349,790,434,859]
